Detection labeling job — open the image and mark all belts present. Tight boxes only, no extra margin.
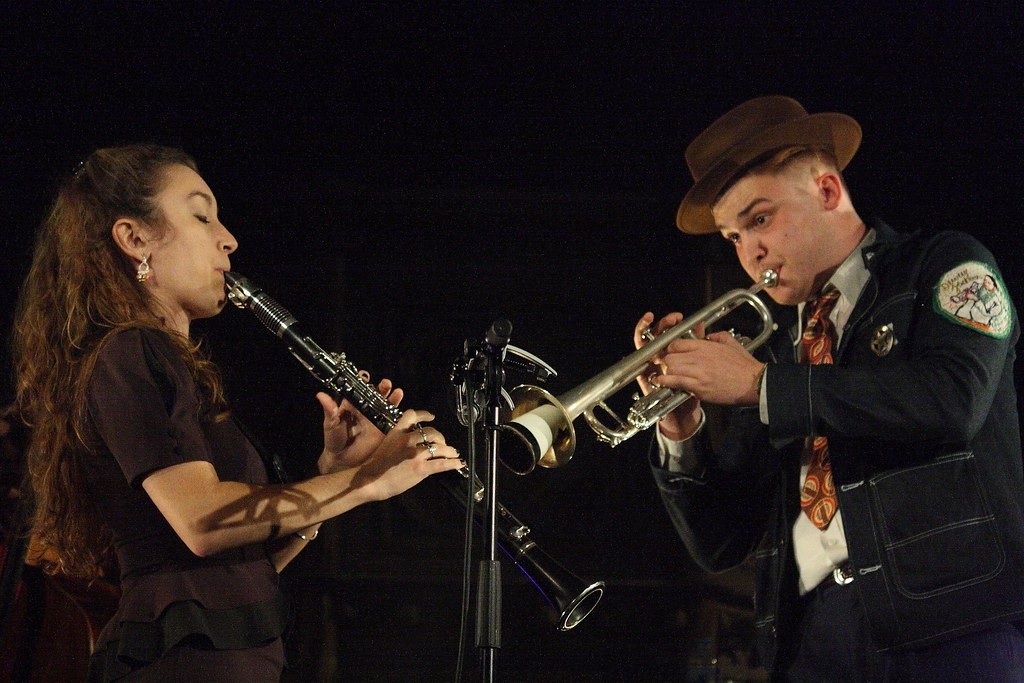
[808,557,851,596]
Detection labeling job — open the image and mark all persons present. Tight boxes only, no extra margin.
[632,97,1024,683]
[12,144,470,682]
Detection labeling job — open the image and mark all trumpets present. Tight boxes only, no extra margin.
[498,270,780,477]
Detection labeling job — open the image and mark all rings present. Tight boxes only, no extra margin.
[424,442,437,460]
[416,428,428,444]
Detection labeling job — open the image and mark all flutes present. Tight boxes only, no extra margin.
[222,270,607,632]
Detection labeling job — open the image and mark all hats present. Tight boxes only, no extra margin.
[673,94,863,234]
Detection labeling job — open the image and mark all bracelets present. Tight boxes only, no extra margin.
[296,531,319,541]
[757,363,769,396]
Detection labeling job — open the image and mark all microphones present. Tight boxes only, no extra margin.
[486,317,513,346]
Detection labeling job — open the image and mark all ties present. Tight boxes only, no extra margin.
[799,287,842,530]
